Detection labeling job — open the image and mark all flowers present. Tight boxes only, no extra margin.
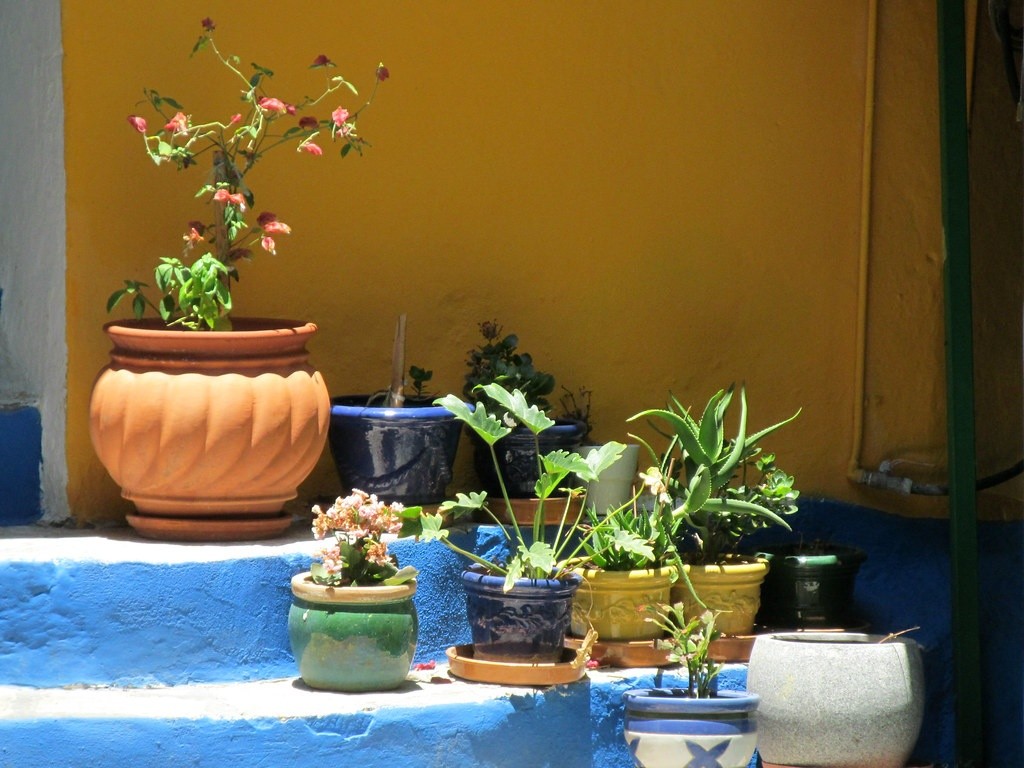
[106,14,390,331]
[309,487,419,587]
[463,319,555,428]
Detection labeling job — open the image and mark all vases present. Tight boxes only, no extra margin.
[746,632,925,768]
[88,315,332,520]
[753,543,861,626]
[287,571,418,692]
[465,419,588,499]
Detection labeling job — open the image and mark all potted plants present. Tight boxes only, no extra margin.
[395,377,800,768]
[328,368,476,508]
